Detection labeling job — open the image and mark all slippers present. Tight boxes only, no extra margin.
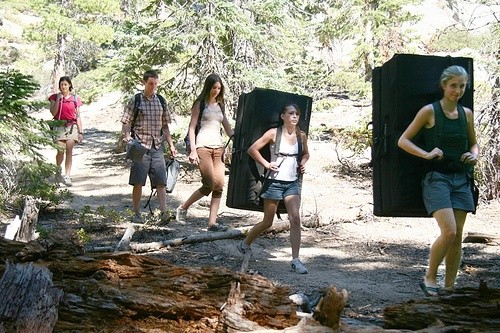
[418,279,438,297]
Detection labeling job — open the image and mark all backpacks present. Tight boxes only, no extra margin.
[184,99,224,154]
[151,155,180,194]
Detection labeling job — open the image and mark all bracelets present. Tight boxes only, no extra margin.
[79,133,82,134]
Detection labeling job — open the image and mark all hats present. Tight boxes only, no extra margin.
[127,140,147,164]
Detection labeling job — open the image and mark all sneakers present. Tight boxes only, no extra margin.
[236,240,258,262]
[55,173,62,182]
[290,258,308,274]
[207,223,229,232]
[175,205,188,226]
[64,176,73,188]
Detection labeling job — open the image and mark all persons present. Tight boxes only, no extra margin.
[398,66,478,296]
[177,74,234,232]
[49,76,83,186]
[120,70,176,220]
[237,104,308,273]
